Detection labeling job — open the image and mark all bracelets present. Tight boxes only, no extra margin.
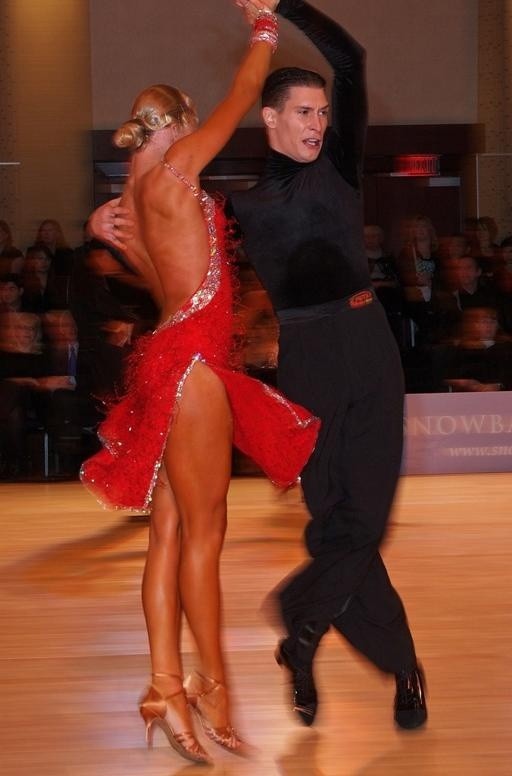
[247,8,280,54]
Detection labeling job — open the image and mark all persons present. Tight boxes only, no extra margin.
[78,0,291,766]
[82,0,430,737]
[1,203,510,488]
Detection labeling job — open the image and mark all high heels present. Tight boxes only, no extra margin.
[138,672,211,764]
[184,675,240,752]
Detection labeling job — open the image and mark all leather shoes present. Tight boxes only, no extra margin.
[394,666,426,730]
[275,639,316,727]
